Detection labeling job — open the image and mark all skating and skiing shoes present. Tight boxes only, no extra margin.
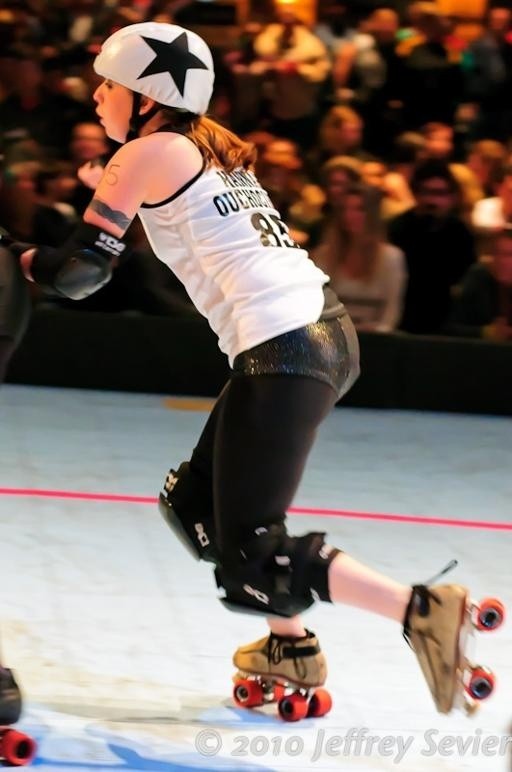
[401,561,503,716]
[233,628,332,723]
[0,667,36,768]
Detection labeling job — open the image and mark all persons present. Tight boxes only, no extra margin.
[0,0,512,343]
[5,21,469,715]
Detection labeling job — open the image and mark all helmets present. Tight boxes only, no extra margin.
[93,21,217,116]
